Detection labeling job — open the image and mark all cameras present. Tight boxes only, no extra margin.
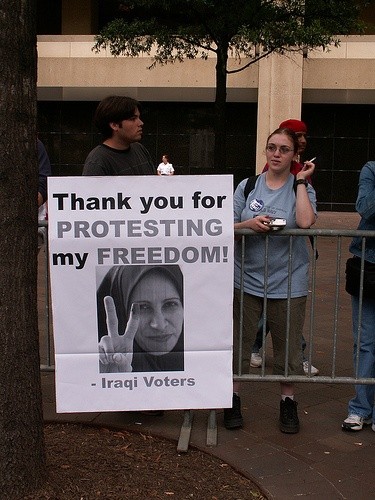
[264,218,287,231]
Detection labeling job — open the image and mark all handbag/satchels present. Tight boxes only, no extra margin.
[344,256,375,299]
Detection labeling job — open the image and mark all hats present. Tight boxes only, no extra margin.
[279,119,307,133]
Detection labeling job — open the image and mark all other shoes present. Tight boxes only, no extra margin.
[249,352,263,368]
[279,397,300,434]
[223,392,243,429]
[302,361,320,376]
[342,414,364,431]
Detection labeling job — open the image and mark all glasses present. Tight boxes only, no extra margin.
[264,144,295,155]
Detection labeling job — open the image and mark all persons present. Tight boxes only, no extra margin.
[82,96,157,176]
[36,137,51,208]
[95,265,183,372]
[341,161,375,432]
[223,127,318,434]
[156,153,174,175]
[249,119,319,375]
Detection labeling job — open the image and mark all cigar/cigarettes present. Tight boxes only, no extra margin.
[304,157,316,166]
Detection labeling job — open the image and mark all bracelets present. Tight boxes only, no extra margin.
[295,178,308,187]
